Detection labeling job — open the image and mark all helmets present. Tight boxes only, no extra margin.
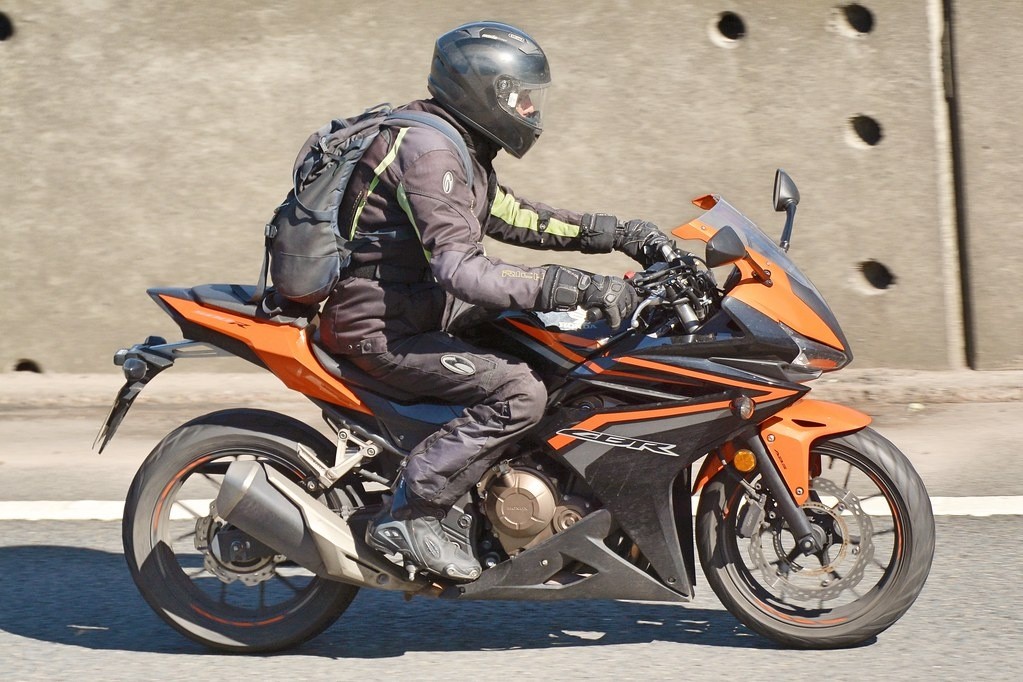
[428,24,551,157]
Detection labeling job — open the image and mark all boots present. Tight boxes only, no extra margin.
[363,469,480,585]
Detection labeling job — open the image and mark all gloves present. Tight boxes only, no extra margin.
[540,261,639,331]
[614,222,674,268]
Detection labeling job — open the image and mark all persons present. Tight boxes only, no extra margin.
[320,20,669,581]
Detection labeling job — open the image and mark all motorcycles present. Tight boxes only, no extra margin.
[92,169,935,654]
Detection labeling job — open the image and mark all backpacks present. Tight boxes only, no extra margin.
[264,100,473,307]
